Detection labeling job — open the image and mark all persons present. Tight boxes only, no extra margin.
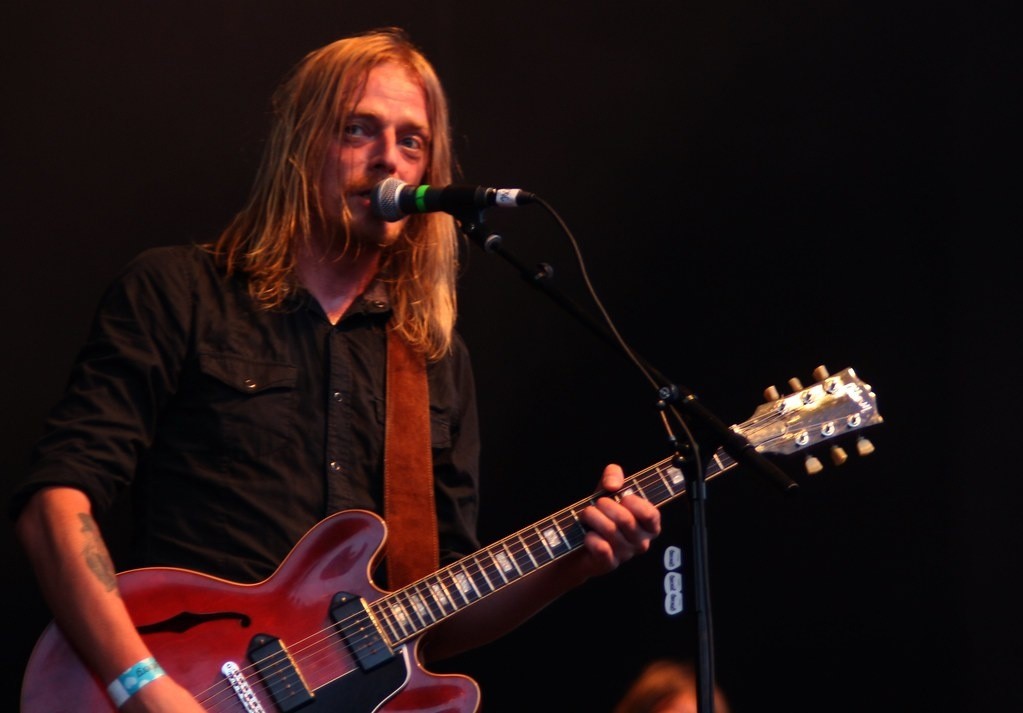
[17,28,665,713]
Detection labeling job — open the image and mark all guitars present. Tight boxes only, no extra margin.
[17,361,887,713]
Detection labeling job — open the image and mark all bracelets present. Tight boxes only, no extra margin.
[107,657,167,706]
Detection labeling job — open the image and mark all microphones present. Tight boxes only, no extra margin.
[370,177,535,223]
